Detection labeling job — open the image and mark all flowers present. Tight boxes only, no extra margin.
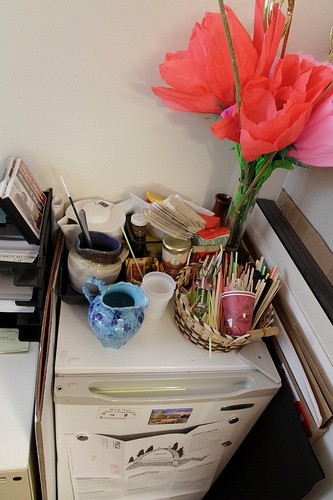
[150,0,333,188]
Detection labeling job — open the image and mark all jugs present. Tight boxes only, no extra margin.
[55,198,144,250]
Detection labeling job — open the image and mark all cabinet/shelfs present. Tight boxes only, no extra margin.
[0,189,53,319]
[55,238,282,500]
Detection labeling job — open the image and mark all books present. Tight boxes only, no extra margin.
[0,157,35,245]
[4,158,48,245]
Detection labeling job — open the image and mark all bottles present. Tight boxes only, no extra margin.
[127,213,148,247]
[161,236,192,276]
[211,192,233,228]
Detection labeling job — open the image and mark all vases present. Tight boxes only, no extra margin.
[218,185,260,253]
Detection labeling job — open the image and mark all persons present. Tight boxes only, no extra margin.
[14,191,31,219]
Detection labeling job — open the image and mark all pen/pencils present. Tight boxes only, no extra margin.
[60,176,120,291]
[175,251,283,353]
[120,224,165,283]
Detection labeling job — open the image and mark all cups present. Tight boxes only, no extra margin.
[137,270,175,325]
[220,291,255,337]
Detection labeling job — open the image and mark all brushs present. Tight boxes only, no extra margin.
[196,226,231,248]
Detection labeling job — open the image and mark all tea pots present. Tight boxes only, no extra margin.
[80,277,150,348]
